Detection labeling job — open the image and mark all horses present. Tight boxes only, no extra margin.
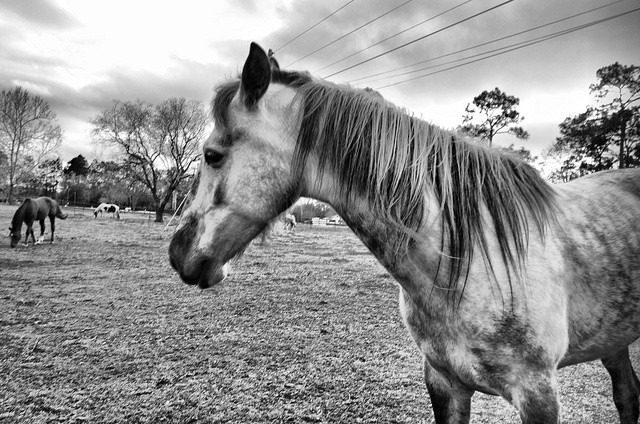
[167,39,640,423]
[7,196,70,248]
[92,202,120,219]
